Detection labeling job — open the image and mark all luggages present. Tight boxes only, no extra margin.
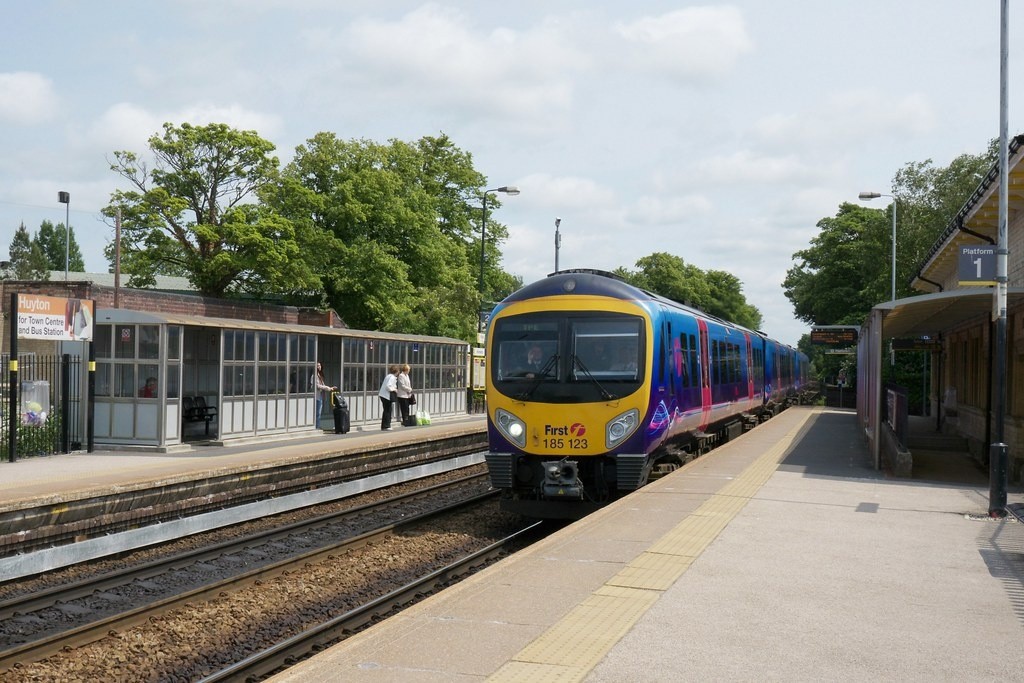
[333,387,350,434]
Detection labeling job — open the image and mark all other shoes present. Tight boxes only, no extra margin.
[381,427,392,430]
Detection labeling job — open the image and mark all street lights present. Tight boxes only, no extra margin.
[59,191,70,282]
[477,185,520,346]
[859,192,897,301]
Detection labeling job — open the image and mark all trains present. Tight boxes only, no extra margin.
[484,267,809,521]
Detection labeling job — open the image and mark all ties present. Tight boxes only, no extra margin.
[623,362,627,371]
[535,361,539,370]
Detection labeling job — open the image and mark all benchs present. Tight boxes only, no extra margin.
[182,396,217,434]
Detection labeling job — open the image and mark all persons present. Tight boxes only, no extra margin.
[138,377,157,398]
[290,367,303,393]
[397,365,412,425]
[512,344,556,378]
[447,369,462,387]
[587,342,611,370]
[378,365,400,431]
[609,347,637,370]
[310,362,337,429]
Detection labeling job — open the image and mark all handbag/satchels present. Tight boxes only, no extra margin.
[409,410,431,425]
[333,392,347,408]
[407,393,416,405]
[390,391,399,401]
